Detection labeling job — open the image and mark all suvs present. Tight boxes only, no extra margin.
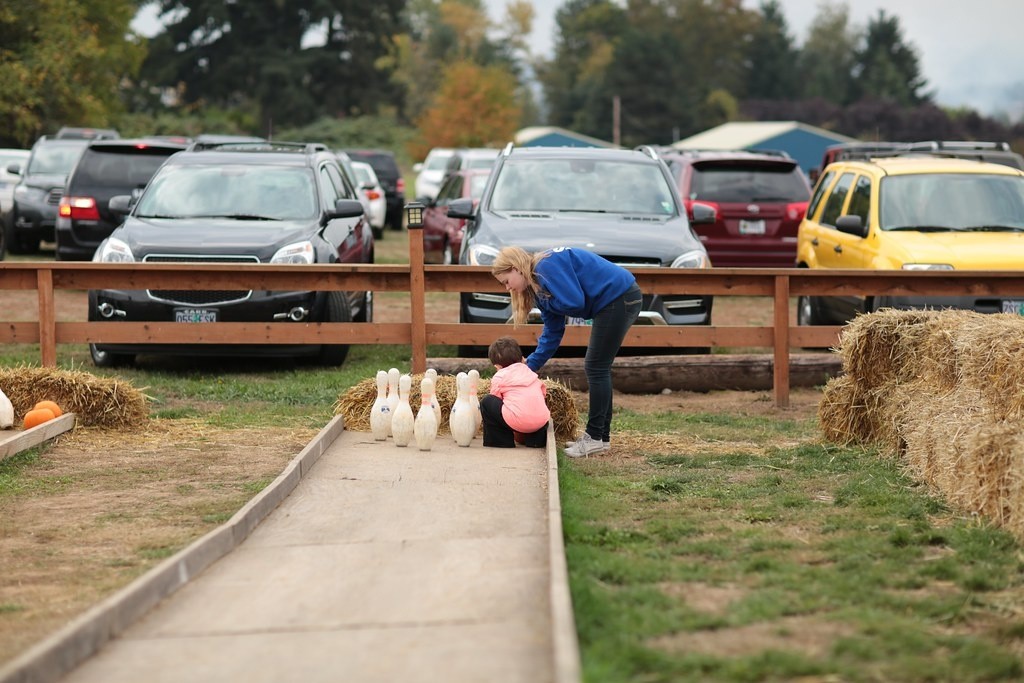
[7,135,120,257]
[86,140,375,367]
[54,142,186,275]
[657,147,813,269]
[339,149,407,230]
[813,141,1024,190]
[445,142,715,360]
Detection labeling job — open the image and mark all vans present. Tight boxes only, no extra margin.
[796,155,1024,352]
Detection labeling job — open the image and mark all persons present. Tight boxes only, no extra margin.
[480,336,550,449]
[492,246,643,457]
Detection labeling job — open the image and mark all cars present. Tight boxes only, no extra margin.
[1,149,32,213]
[349,162,386,240]
[422,151,500,266]
[411,148,454,200]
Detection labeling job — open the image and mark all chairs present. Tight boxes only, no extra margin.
[100,162,128,183]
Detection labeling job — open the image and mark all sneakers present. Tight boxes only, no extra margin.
[565,442,610,450]
[563,432,603,457]
